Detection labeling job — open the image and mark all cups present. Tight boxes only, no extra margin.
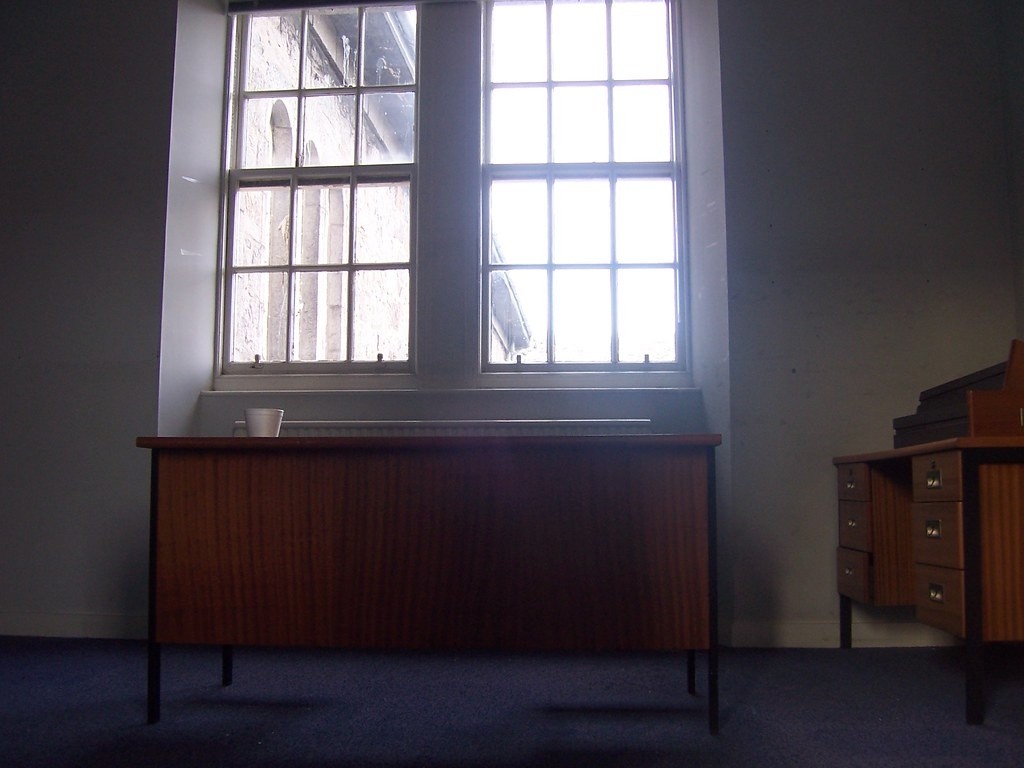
[244,408,284,437]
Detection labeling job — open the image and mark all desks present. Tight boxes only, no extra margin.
[136,434,723,741]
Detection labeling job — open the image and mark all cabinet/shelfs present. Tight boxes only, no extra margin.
[832,438,1024,727]
